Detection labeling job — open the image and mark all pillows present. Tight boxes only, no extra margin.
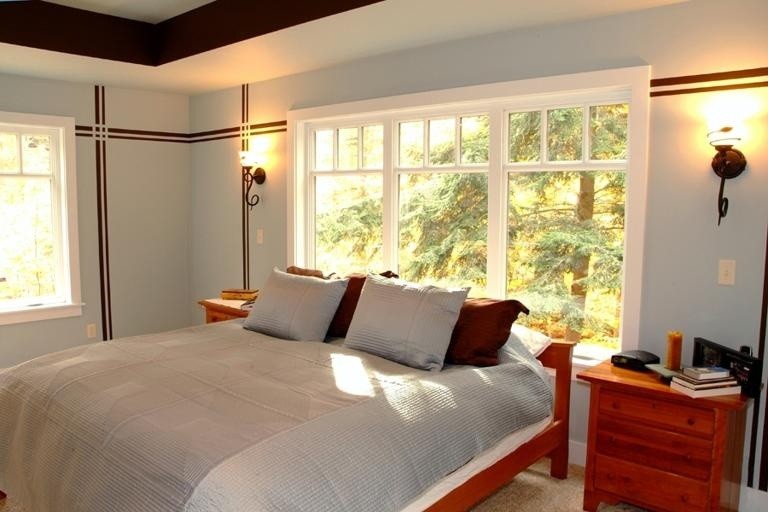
[244,265,528,373]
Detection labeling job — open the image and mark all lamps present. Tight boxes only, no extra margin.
[240,135,273,212]
[699,93,758,226]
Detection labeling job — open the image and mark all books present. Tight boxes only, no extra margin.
[671,374,737,389]
[682,365,730,380]
[668,381,741,399]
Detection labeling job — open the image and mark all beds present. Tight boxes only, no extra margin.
[0,315,577,512]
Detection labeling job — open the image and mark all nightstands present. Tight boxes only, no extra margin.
[576,352,764,511]
[198,293,257,324]
